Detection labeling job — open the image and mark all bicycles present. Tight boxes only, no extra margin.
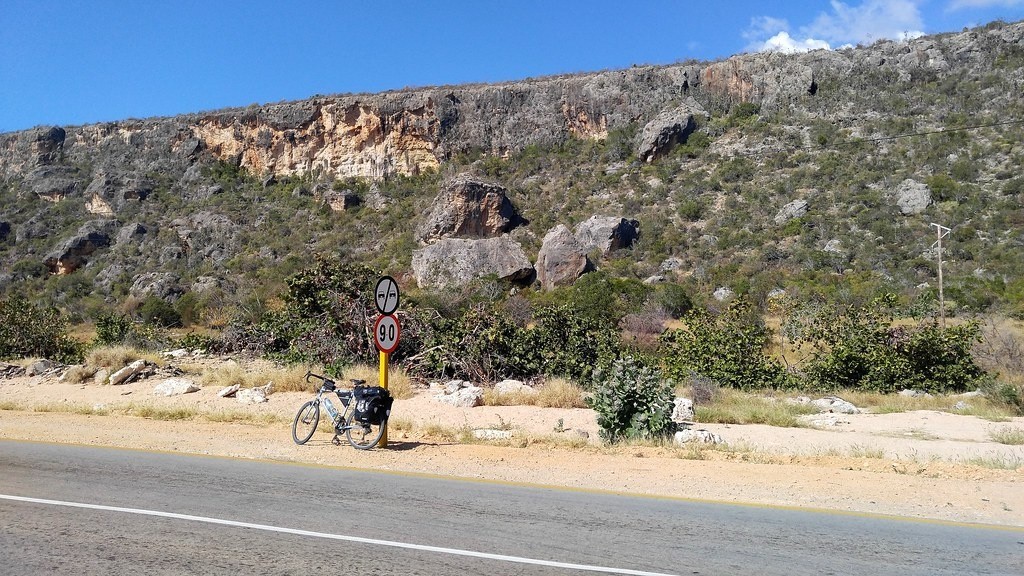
[291,371,385,450]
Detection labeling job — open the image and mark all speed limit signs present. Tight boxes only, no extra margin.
[374,314,400,353]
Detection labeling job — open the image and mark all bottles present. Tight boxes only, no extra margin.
[324,397,337,416]
